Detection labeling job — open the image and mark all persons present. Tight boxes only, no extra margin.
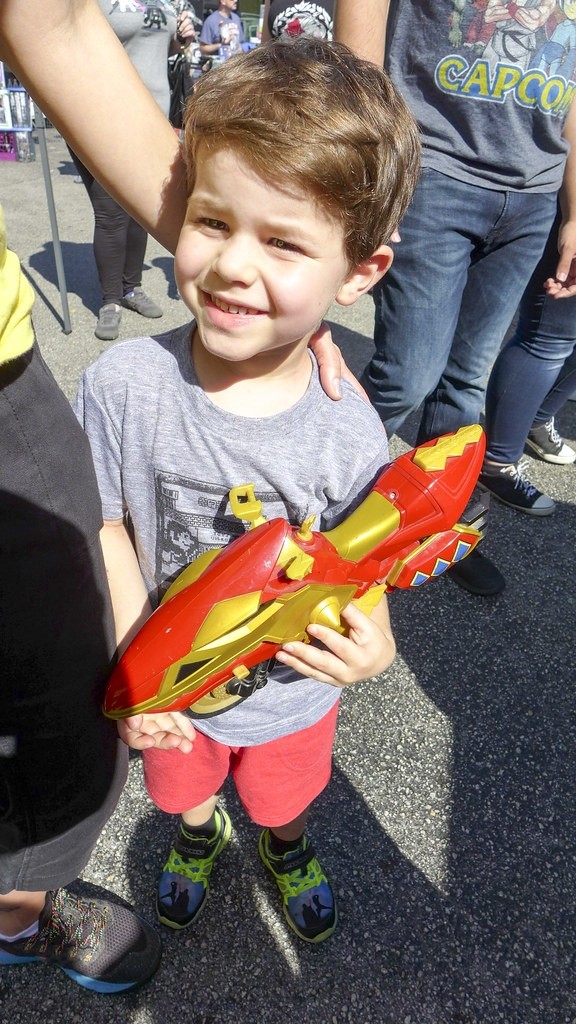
[0,0,371,994]
[73,40,422,943]
[67,0,576,596]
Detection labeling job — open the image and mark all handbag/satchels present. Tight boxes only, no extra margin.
[167,57,195,129]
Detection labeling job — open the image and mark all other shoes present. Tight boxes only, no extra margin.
[446,549,506,596]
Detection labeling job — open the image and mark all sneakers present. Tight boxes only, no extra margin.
[525,415,576,465]
[476,460,556,516]
[94,303,121,340]
[122,286,162,317]
[258,825,337,943]
[0,887,163,995]
[156,805,231,929]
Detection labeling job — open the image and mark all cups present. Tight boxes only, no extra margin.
[219,45,231,64]
